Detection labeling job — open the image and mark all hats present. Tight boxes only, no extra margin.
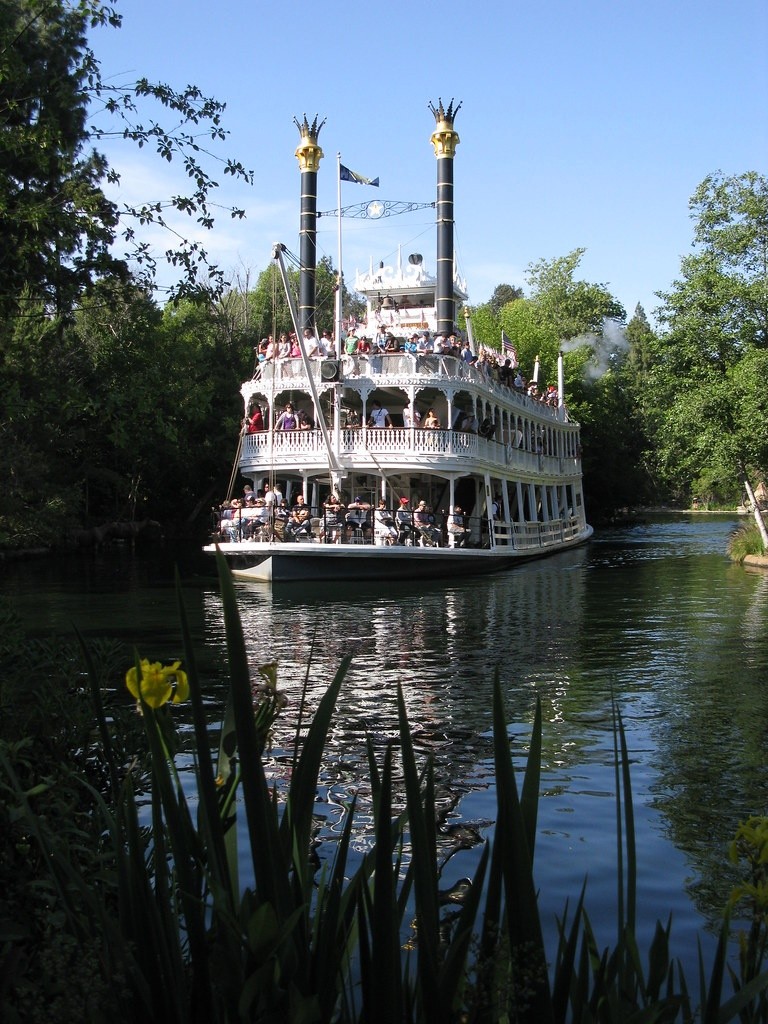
[236,499,243,503]
[361,336,367,340]
[522,376,526,381]
[399,498,410,504]
[467,412,475,417]
[257,498,266,506]
[243,485,250,490]
[348,327,356,332]
[418,500,428,506]
[355,496,363,502]
[378,323,387,327]
[259,338,269,344]
[297,409,304,416]
[423,331,430,336]
[244,496,255,502]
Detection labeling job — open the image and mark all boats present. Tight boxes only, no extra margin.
[200,97,595,584]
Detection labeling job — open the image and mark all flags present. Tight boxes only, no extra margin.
[350,313,356,325]
[339,162,380,187]
[389,309,427,323]
[503,332,518,355]
[341,320,349,330]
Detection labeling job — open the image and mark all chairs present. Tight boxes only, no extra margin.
[345,513,364,545]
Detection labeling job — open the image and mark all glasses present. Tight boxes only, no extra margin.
[285,405,291,408]
[323,335,327,337]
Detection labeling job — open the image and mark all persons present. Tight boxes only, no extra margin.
[240,323,583,460]
[400,295,412,305]
[208,482,504,549]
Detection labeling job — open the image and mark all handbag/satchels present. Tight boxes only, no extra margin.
[290,414,296,429]
[369,421,376,427]
